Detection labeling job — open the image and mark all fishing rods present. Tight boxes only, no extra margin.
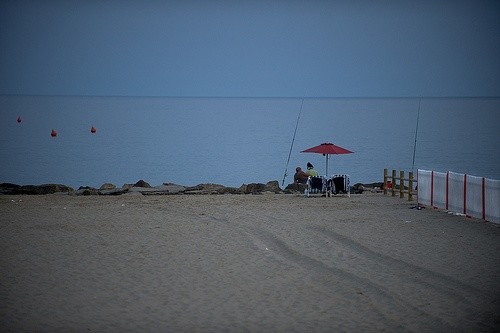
[281,95,305,186]
[412,98,422,174]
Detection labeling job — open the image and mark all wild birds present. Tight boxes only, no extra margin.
[51,128,57,136]
[17,115,22,123]
[91,125,96,133]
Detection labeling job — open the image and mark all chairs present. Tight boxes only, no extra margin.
[304,175,350,198]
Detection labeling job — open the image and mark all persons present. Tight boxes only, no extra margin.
[294,167,309,184]
[307,162,318,176]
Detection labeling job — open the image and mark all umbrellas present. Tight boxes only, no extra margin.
[301,143,354,180]
